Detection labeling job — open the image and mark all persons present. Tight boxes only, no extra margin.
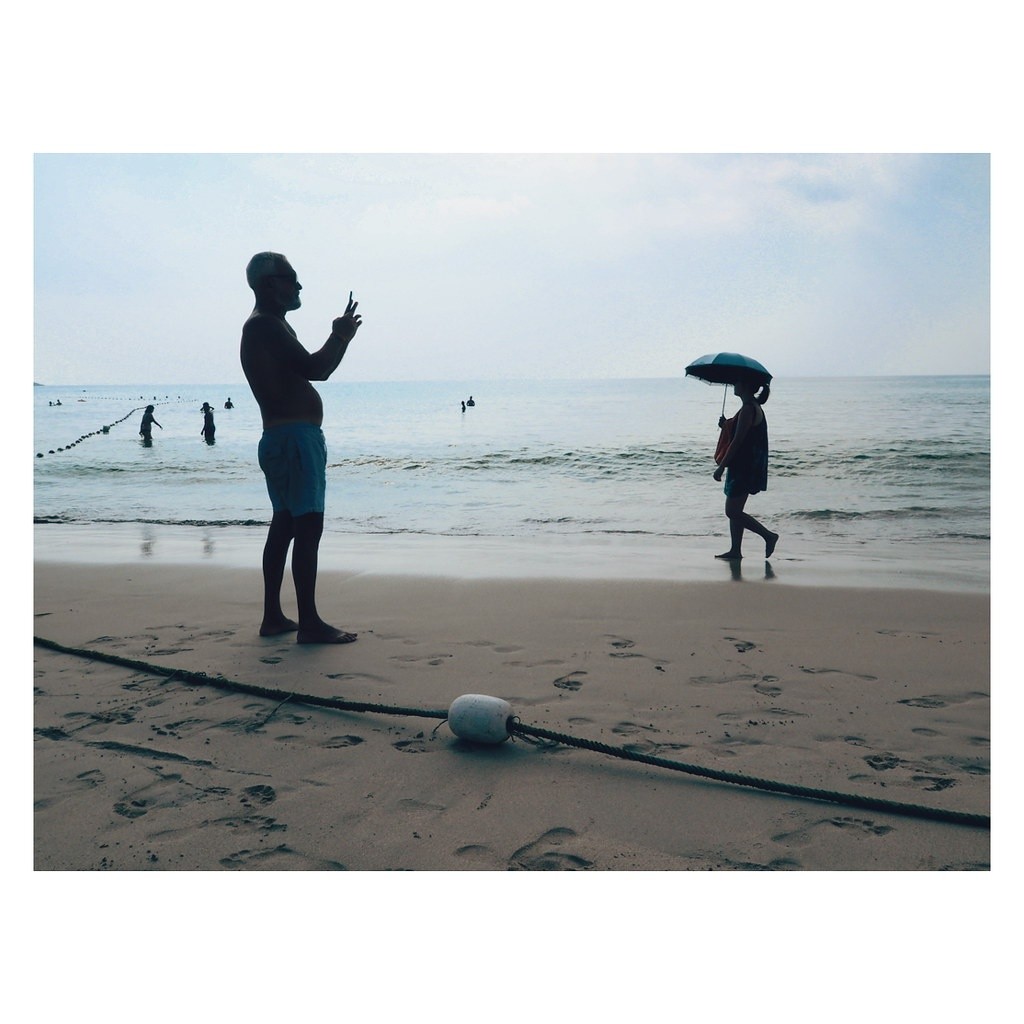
[139,405,162,443]
[49,399,62,406]
[200,402,216,445]
[240,251,363,647]
[713,371,779,559]
[461,396,475,413]
[224,398,234,409]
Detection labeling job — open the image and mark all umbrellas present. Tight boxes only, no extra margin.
[684,352,773,425]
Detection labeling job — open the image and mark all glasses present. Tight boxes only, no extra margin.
[268,270,296,283]
